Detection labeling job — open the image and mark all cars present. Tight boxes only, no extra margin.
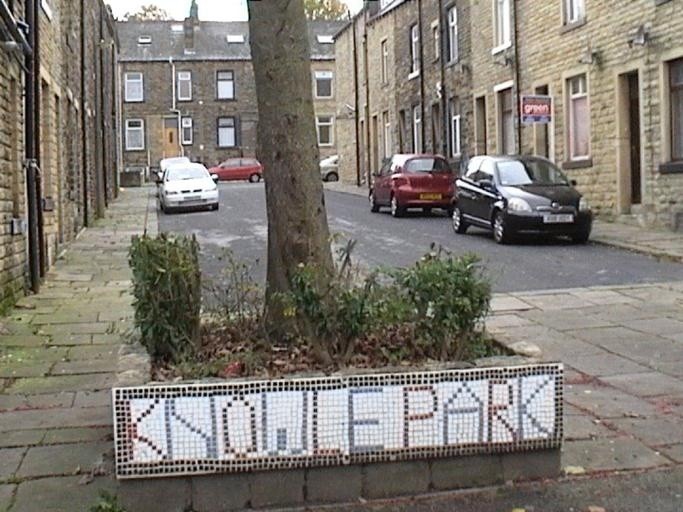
[152,156,220,215]
[451,153,594,243]
[208,155,264,183]
[369,153,458,218]
[319,154,340,182]
